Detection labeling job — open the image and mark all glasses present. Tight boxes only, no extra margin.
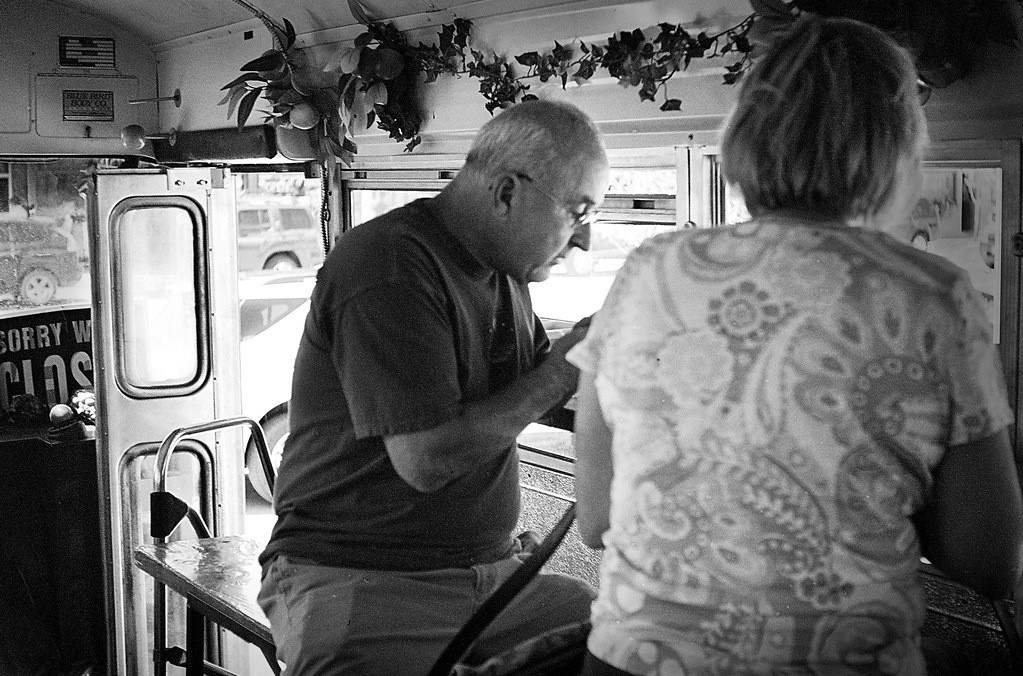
[917,79,933,108]
[489,171,600,229]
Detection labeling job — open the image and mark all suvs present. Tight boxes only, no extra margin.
[236,189,326,277]
[0,211,85,310]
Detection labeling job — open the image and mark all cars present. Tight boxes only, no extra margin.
[237,266,320,505]
[900,166,1000,271]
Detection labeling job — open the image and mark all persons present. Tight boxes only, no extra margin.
[572,11,1023,676]
[259,99,612,676]
